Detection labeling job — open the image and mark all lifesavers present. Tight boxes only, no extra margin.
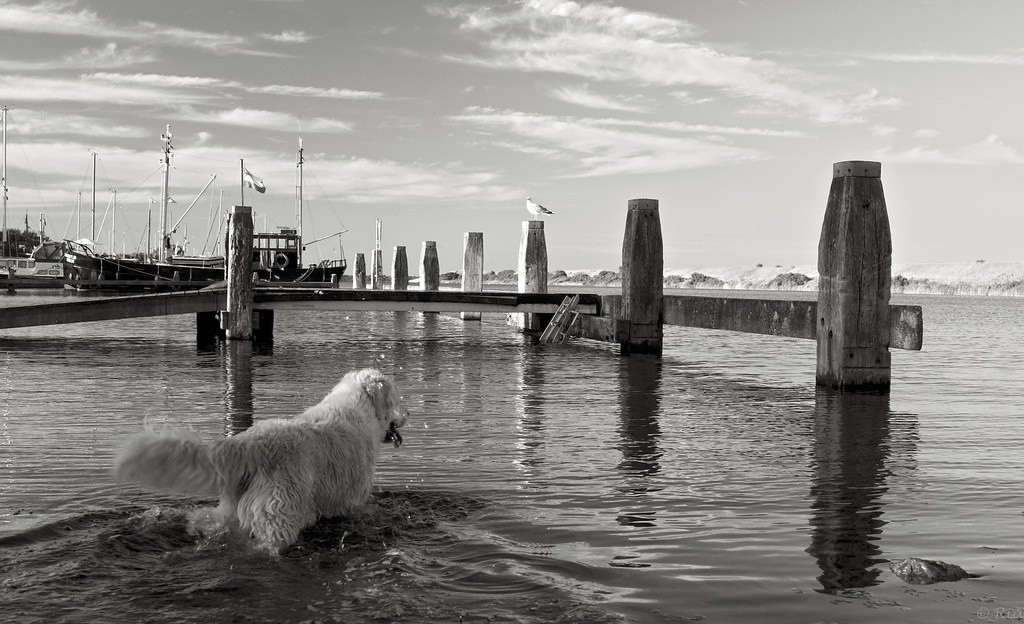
[274,253,290,269]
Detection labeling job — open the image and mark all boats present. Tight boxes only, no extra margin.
[0,104,227,289]
[56,122,350,293]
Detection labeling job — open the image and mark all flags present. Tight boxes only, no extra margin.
[243,167,267,194]
[151,197,157,204]
[167,195,176,203]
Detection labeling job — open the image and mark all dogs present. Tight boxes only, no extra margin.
[119,368,412,552]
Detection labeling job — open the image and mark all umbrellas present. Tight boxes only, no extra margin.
[74,238,104,245]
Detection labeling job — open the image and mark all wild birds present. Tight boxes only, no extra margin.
[526,196,556,221]
